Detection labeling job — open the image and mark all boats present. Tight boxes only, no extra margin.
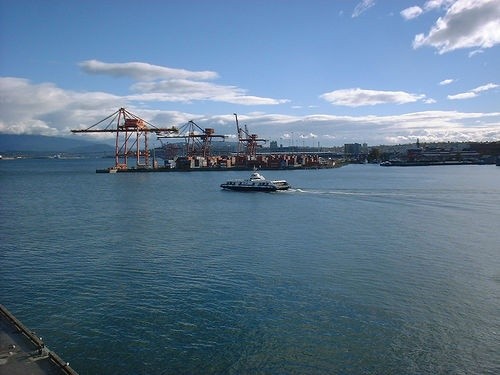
[379,137,484,167]
[220,164,292,192]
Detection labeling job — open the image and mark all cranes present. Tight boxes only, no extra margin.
[156,119,228,159]
[71,107,178,171]
[231,113,267,159]
